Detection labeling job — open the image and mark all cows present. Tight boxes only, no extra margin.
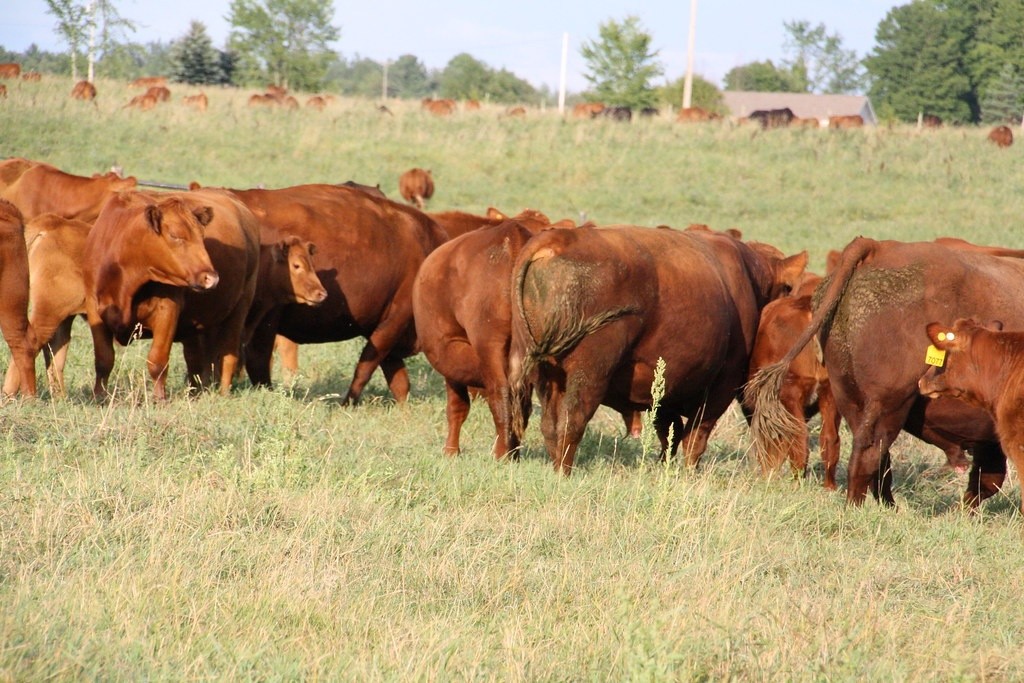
[1,61,1024,516]
